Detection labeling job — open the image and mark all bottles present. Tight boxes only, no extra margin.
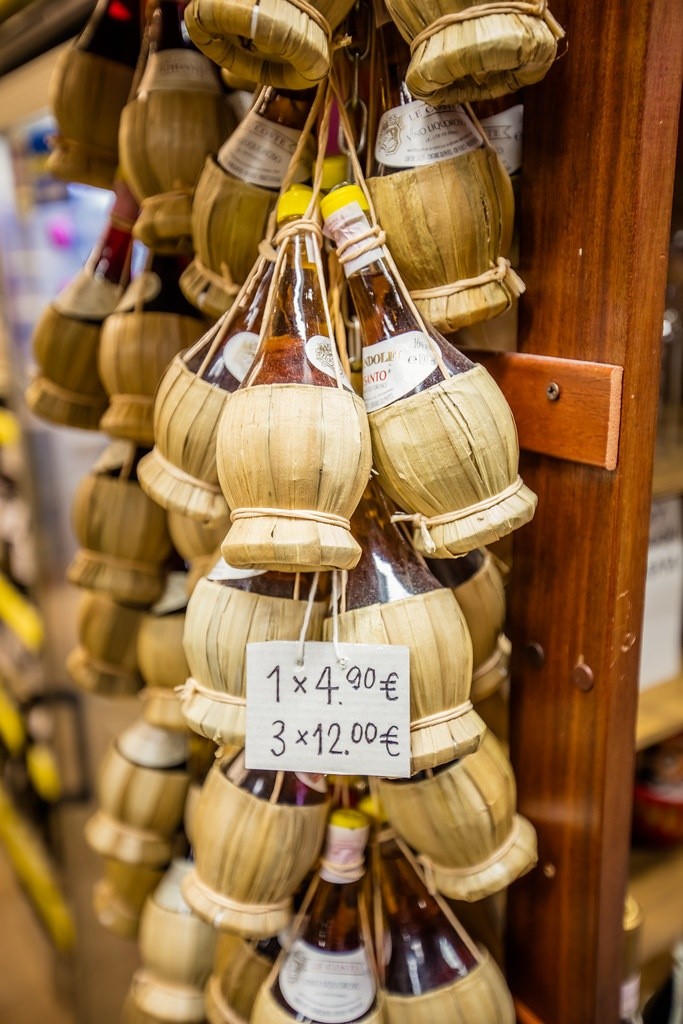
[25,0,559,1024]
